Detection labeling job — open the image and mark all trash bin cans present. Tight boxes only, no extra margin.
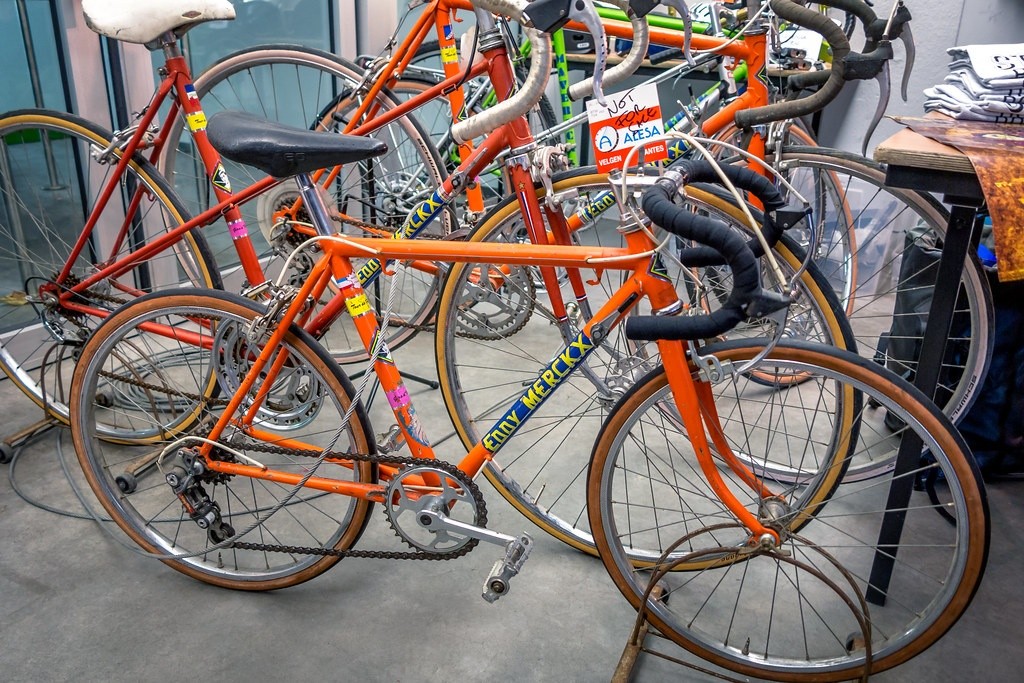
[882,225,1024,439]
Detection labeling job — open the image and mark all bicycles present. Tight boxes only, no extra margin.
[68,111,994,683]
[0,1,996,488]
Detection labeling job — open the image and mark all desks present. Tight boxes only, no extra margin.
[858,101,1024,610]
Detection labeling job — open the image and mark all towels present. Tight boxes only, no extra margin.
[922,42,1024,125]
